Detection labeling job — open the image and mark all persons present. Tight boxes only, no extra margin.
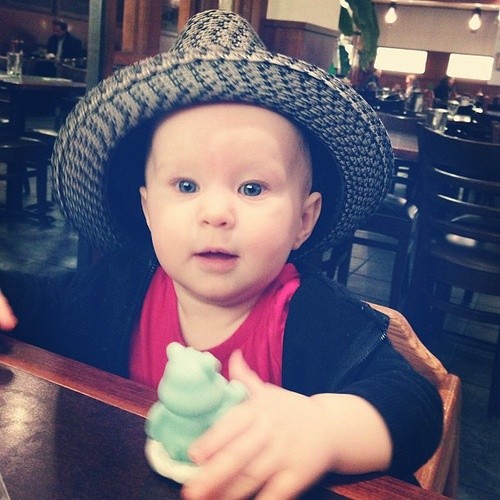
[45,19,81,60]
[357,61,458,114]
[1,10,445,500]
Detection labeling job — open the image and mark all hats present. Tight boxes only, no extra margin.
[51,9,397,274]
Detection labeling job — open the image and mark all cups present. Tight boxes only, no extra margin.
[7,53,23,84]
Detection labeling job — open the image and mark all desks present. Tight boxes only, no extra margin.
[0,333,455,500]
[386,127,419,161]
[1,70,87,217]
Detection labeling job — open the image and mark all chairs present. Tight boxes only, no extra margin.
[0,64,500,418]
